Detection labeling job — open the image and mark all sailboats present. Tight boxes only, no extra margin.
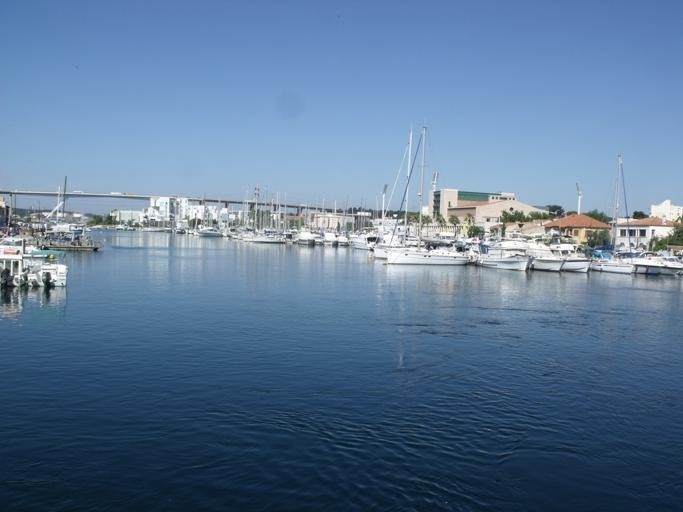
[197,189,373,251]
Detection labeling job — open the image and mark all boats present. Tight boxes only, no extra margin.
[1,222,101,289]
